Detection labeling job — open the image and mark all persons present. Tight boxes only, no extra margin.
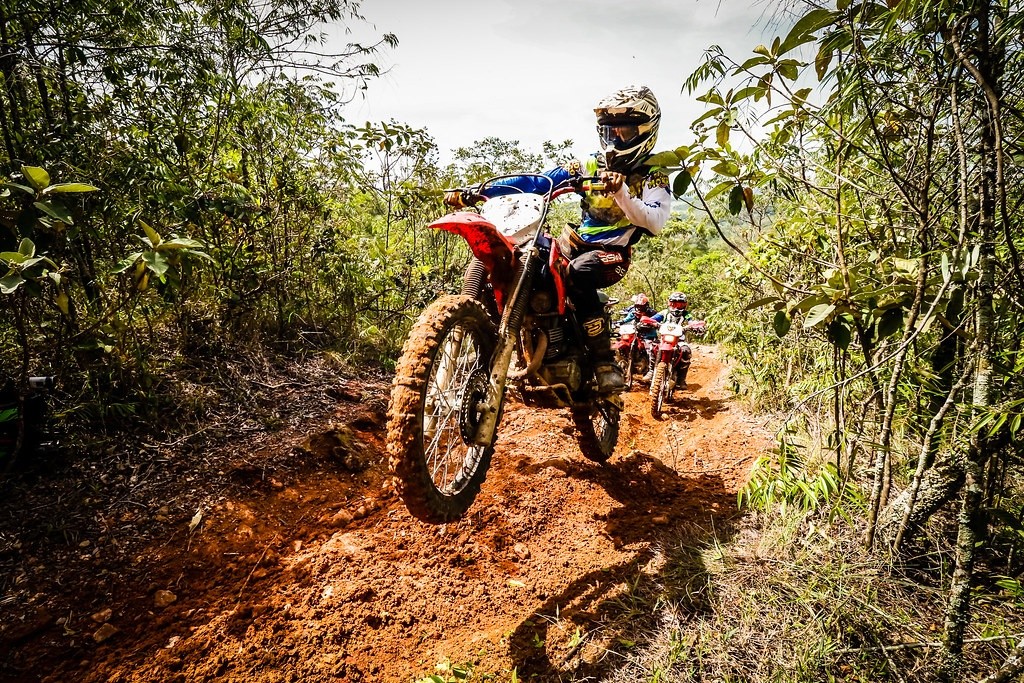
[614,292,656,383]
[637,292,705,391]
[438,83,672,395]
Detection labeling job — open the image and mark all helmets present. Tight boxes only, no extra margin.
[595,87,661,170]
[634,293,649,312]
[668,292,688,317]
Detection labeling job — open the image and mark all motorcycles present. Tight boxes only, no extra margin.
[611,319,660,392]
[640,316,705,419]
[386,162,630,525]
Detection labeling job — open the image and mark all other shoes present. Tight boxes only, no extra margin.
[642,369,654,383]
[678,379,687,389]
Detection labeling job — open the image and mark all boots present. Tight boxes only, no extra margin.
[578,313,629,392]
